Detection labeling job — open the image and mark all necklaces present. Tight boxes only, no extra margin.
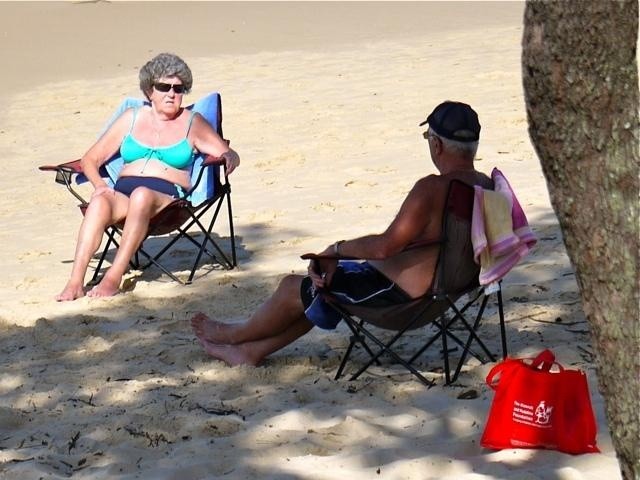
[148,108,174,139]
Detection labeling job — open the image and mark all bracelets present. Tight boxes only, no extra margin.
[333,239,350,257]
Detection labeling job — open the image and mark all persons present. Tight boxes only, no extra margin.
[191,100,497,366]
[53,54,241,302]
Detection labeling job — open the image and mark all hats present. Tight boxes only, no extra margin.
[419,102,481,141]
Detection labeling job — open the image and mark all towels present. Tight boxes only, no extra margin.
[469,169,538,295]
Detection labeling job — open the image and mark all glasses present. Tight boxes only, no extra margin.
[153,82,184,94]
[423,132,442,144]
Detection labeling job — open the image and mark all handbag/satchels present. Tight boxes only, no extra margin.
[481,356,600,457]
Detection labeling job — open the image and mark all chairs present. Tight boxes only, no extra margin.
[324,178,507,388]
[38,92,237,284]
